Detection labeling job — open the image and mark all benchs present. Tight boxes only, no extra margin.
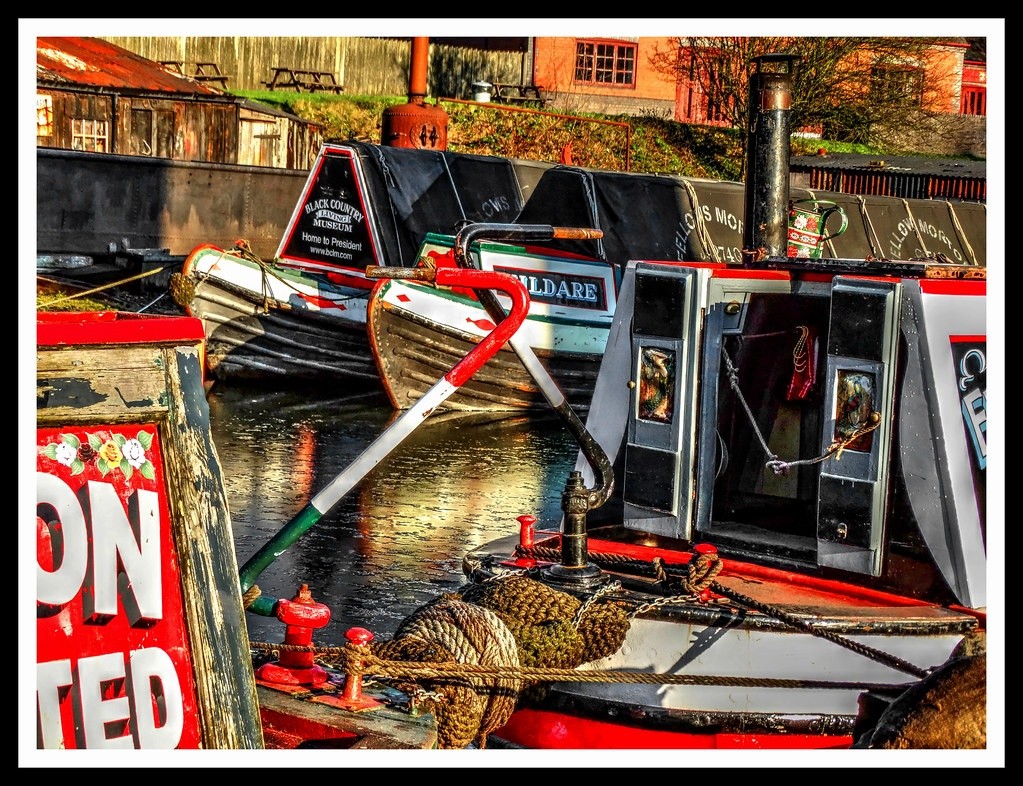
[301,83,344,91]
[260,81,307,88]
[189,74,233,82]
[501,96,545,103]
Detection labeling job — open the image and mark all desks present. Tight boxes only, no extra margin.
[156,60,230,90]
[270,68,343,95]
[492,82,546,110]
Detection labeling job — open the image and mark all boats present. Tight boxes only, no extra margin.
[37,42,985,752]
